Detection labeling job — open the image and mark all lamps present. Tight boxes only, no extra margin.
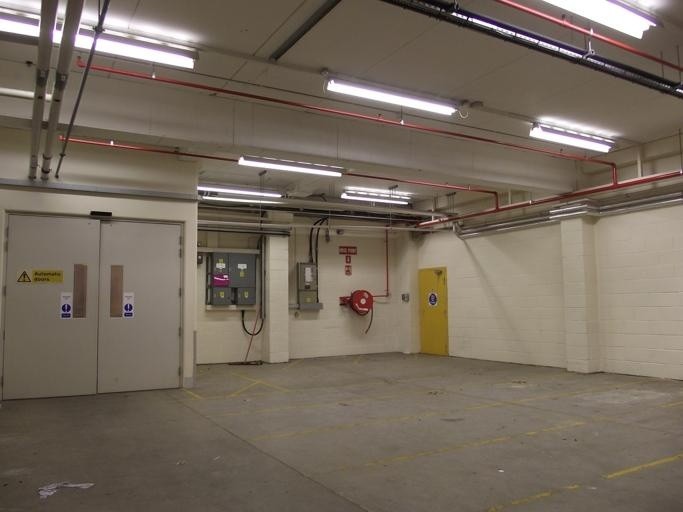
[0,7,200,73]
[197,154,411,205]
[529,122,617,156]
[545,0,660,40]
[319,68,459,119]
